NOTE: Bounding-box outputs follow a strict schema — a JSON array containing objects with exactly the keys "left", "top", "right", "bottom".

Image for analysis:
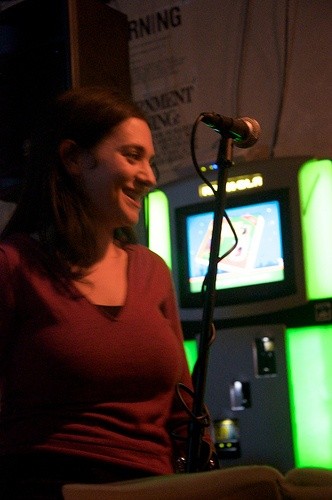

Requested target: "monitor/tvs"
[{"left": 174, "top": 186, "right": 296, "bottom": 309}]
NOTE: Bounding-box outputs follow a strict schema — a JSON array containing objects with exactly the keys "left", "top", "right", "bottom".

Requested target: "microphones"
[{"left": 199, "top": 111, "right": 261, "bottom": 148}]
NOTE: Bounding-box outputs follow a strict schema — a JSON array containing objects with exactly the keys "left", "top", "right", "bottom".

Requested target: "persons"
[{"left": 0, "top": 90, "right": 213, "bottom": 497}]
